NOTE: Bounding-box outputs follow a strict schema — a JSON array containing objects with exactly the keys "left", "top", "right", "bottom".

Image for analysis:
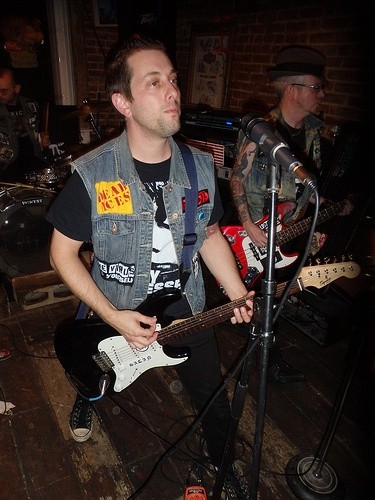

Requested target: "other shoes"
[{"left": 268, "top": 360, "right": 306, "bottom": 382}]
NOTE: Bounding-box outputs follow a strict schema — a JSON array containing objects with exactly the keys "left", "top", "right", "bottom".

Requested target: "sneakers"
[
  {"left": 224, "top": 468, "right": 249, "bottom": 500},
  {"left": 69, "top": 398, "right": 94, "bottom": 442}
]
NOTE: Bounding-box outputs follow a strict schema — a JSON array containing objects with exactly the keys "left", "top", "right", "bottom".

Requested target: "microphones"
[{"left": 241, "top": 113, "right": 313, "bottom": 187}]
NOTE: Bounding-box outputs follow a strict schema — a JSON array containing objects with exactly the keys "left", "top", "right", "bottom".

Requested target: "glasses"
[{"left": 292, "top": 82, "right": 322, "bottom": 93}]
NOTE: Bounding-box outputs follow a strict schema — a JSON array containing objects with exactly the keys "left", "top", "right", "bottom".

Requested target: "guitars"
[
  {"left": 55, "top": 253, "right": 363, "bottom": 399},
  {"left": 211, "top": 197, "right": 352, "bottom": 297}
]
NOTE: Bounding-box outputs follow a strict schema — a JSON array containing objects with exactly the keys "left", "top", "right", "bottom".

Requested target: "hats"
[{"left": 266, "top": 46, "right": 329, "bottom": 86}]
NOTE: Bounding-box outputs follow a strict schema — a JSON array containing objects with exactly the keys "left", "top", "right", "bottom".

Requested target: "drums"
[
  {"left": 0, "top": 185, "right": 61, "bottom": 280},
  {"left": 24, "top": 159, "right": 76, "bottom": 185}
]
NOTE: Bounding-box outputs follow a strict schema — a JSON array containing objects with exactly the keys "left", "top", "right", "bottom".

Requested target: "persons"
[
  {"left": 0, "top": 69, "right": 59, "bottom": 180},
  {"left": 233, "top": 62, "right": 361, "bottom": 383},
  {"left": 47, "top": 35, "right": 252, "bottom": 500}
]
